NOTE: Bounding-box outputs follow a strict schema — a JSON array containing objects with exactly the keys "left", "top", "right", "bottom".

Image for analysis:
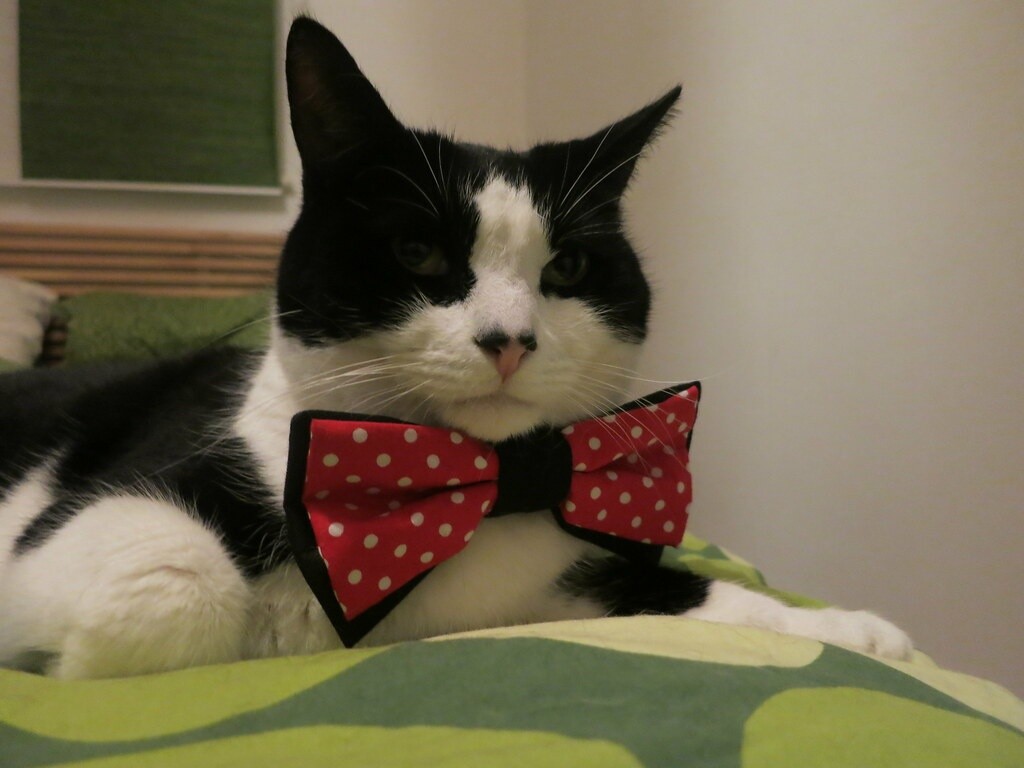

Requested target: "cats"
[{"left": 0, "top": 15, "right": 914, "bottom": 681}]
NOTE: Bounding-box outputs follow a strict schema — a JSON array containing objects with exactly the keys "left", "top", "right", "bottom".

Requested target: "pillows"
[{"left": 0, "top": 271, "right": 278, "bottom": 369}]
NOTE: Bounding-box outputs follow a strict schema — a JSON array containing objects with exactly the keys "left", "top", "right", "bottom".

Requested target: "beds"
[{"left": 0, "top": 222, "right": 1024, "bottom": 766}]
